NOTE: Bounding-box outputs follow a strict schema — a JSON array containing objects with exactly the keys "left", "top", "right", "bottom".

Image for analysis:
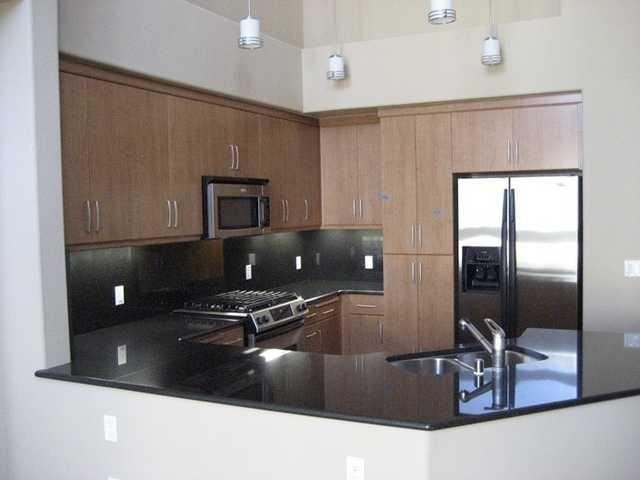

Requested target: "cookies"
[{"left": 201, "top": 175, "right": 272, "bottom": 241}]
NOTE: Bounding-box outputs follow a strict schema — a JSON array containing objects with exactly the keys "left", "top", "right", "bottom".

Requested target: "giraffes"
[{"left": 172, "top": 289, "right": 309, "bottom": 334}]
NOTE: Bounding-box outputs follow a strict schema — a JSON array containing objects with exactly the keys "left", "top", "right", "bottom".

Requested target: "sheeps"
[
  {"left": 459, "top": 371, "right": 509, "bottom": 410},
  {"left": 459, "top": 316, "right": 507, "bottom": 369}
]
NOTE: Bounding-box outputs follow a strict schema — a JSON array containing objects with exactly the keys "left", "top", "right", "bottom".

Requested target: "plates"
[
  {"left": 453, "top": 103, "right": 582, "bottom": 172},
  {"left": 261, "top": 112, "right": 296, "bottom": 232},
  {"left": 126, "top": 83, "right": 203, "bottom": 241},
  {"left": 203, "top": 99, "right": 261, "bottom": 180},
  {"left": 60, "top": 72, "right": 125, "bottom": 245},
  {"left": 381, "top": 256, "right": 456, "bottom": 350},
  {"left": 306, "top": 296, "right": 342, "bottom": 356},
  {"left": 321, "top": 125, "right": 383, "bottom": 227},
  {"left": 191, "top": 326, "right": 243, "bottom": 348},
  {"left": 382, "top": 113, "right": 455, "bottom": 256},
  {"left": 342, "top": 294, "right": 383, "bottom": 352}
]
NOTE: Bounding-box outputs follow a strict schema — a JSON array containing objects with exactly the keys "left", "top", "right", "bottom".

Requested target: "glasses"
[
  {"left": 479, "top": 0, "right": 503, "bottom": 69},
  {"left": 428, "top": 0, "right": 458, "bottom": 31},
  {"left": 326, "top": 0, "right": 347, "bottom": 81},
  {"left": 236, "top": 0, "right": 265, "bottom": 51}
]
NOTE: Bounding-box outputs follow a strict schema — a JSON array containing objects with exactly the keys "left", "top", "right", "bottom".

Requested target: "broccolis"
[
  {"left": 452, "top": 169, "right": 581, "bottom": 344},
  {"left": 454, "top": 344, "right": 581, "bottom": 410}
]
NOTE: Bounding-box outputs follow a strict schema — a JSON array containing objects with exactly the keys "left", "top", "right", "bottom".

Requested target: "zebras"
[{"left": 255, "top": 319, "right": 307, "bottom": 354}]
